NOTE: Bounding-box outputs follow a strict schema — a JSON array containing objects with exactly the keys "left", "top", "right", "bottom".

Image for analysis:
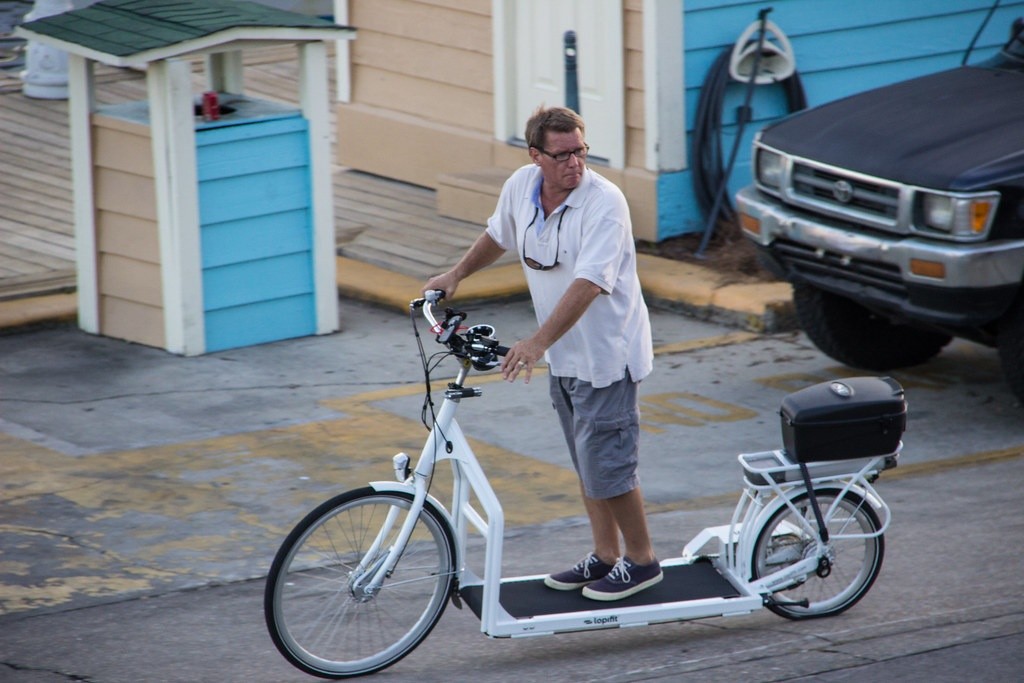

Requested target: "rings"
[{"left": 518, "top": 361, "right": 524, "bottom": 366}]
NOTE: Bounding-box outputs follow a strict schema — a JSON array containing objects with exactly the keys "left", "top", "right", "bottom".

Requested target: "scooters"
[{"left": 260, "top": 288, "right": 910, "bottom": 683}]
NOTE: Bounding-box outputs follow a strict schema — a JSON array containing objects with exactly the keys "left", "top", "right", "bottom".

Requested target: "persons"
[{"left": 423, "top": 106, "right": 665, "bottom": 600}]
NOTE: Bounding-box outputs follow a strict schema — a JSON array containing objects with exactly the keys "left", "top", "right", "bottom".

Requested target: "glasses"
[
  {"left": 529, "top": 143, "right": 589, "bottom": 162},
  {"left": 523, "top": 221, "right": 559, "bottom": 271}
]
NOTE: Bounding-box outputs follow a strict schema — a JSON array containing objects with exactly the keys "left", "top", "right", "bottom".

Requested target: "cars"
[{"left": 733, "top": 13, "right": 1024, "bottom": 402}]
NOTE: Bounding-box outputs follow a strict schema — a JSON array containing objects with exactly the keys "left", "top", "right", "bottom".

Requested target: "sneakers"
[
  {"left": 544, "top": 551, "right": 623, "bottom": 590},
  {"left": 582, "top": 555, "right": 664, "bottom": 601}
]
